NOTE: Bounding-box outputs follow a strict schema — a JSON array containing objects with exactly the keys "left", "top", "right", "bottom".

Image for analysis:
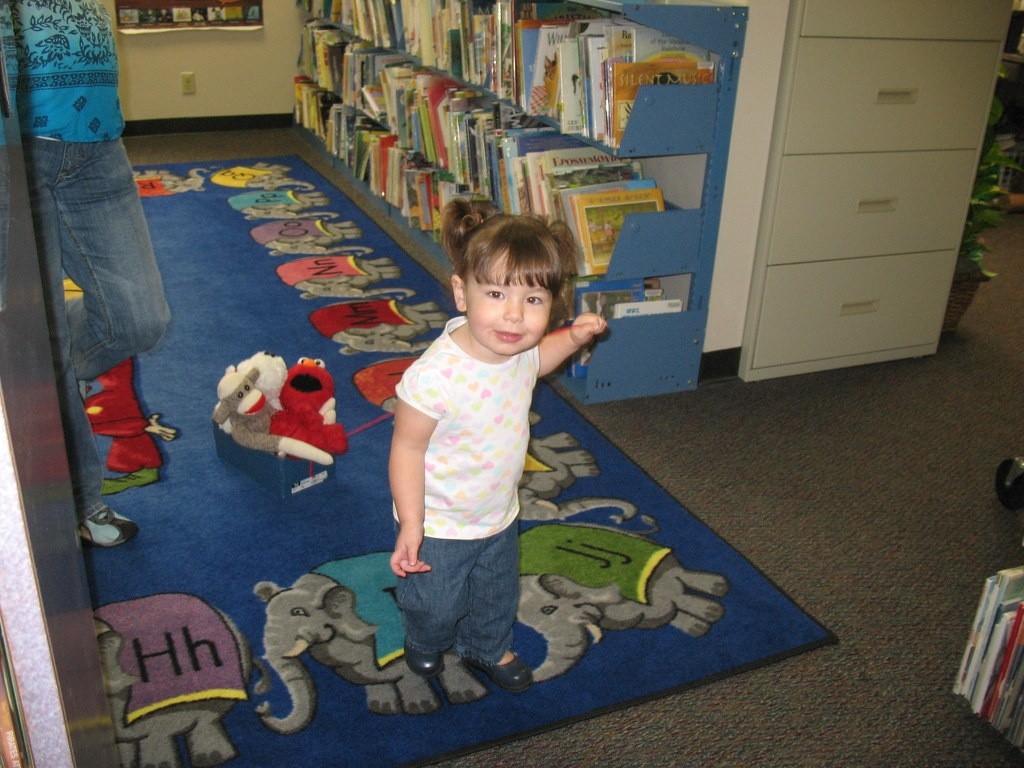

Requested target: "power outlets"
[{"left": 180, "top": 70, "right": 195, "bottom": 94}]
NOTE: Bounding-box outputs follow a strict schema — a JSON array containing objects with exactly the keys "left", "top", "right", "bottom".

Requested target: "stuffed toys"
[{"left": 216, "top": 349, "right": 349, "bottom": 465}]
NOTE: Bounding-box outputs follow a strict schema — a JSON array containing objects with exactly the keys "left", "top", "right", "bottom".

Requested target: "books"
[
  {"left": 293, "top": 75, "right": 475, "bottom": 243},
  {"left": 296, "top": 1, "right": 718, "bottom": 149},
  {"left": 445, "top": 109, "right": 683, "bottom": 379},
  {"left": 117, "top": 6, "right": 259, "bottom": 24}
]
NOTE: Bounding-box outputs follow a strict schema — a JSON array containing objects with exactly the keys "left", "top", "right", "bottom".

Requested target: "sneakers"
[{"left": 78, "top": 507, "right": 140, "bottom": 546}]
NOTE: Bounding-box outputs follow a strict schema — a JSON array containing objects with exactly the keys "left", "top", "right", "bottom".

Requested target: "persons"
[
  {"left": 387, "top": 200, "right": 608, "bottom": 693},
  {"left": 0, "top": 1, "right": 173, "bottom": 547}
]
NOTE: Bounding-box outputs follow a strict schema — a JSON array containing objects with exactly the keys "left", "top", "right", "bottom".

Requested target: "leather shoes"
[
  {"left": 461, "top": 650, "right": 534, "bottom": 692},
  {"left": 404, "top": 636, "right": 445, "bottom": 678}
]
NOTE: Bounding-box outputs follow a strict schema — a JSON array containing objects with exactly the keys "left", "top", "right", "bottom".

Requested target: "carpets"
[{"left": 63, "top": 152, "right": 846, "bottom": 768}]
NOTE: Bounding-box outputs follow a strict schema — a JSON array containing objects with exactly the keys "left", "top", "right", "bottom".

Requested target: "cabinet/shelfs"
[{"left": 288, "top": 0, "right": 1017, "bottom": 406}]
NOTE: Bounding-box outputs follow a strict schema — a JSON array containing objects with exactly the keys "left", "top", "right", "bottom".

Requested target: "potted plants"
[{"left": 938, "top": 63, "right": 1024, "bottom": 332}]
